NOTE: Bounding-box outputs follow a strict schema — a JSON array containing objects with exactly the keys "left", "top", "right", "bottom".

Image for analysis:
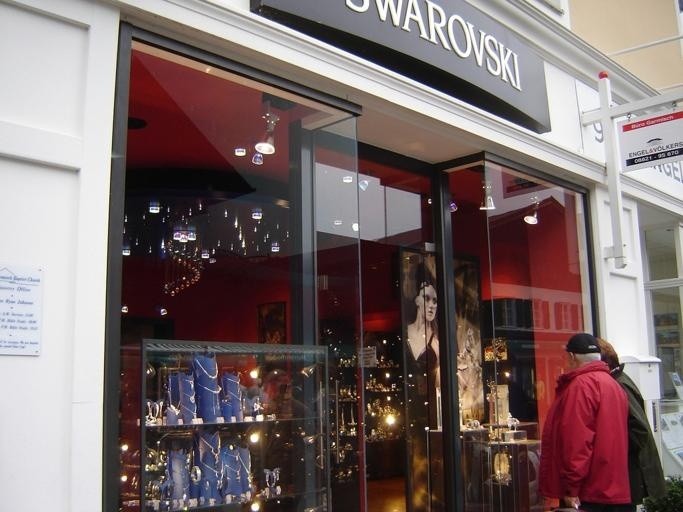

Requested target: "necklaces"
[{"left": 161, "top": 357, "right": 257, "bottom": 485}]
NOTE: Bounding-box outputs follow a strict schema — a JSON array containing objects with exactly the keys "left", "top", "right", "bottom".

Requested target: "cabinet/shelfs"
[
  {"left": 118, "top": 338, "right": 332, "bottom": 512},
  {"left": 364, "top": 332, "right": 407, "bottom": 480}
]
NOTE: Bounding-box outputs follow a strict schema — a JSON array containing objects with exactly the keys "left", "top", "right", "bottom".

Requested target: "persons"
[
  {"left": 408, "top": 272, "right": 446, "bottom": 461},
  {"left": 538, "top": 332, "right": 636, "bottom": 511},
  {"left": 594, "top": 337, "right": 664, "bottom": 512}
]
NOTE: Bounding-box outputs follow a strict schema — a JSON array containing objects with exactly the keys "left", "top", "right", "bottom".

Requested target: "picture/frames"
[{"left": 257, "top": 301, "right": 286, "bottom": 370}]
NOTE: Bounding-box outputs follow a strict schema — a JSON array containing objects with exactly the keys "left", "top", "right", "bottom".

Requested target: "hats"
[{"left": 566, "top": 333, "right": 601, "bottom": 353}]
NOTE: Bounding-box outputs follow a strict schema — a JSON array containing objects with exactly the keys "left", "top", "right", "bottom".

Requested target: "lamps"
[
  {"left": 524, "top": 194, "right": 540, "bottom": 225},
  {"left": 255, "top": 90, "right": 296, "bottom": 156},
  {"left": 468, "top": 164, "right": 496, "bottom": 210}
]
